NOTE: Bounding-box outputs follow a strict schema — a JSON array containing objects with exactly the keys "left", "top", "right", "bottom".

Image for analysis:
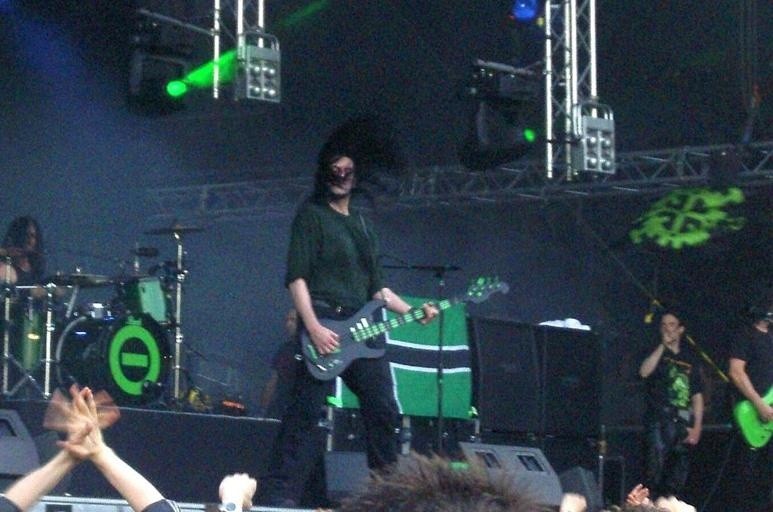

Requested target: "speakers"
[
  {"left": 458, "top": 440, "right": 564, "bottom": 507},
  {"left": 469, "top": 316, "right": 540, "bottom": 435},
  {"left": 0, "top": 408, "right": 41, "bottom": 477},
  {"left": 561, "top": 467, "right": 603, "bottom": 511},
  {"left": 536, "top": 324, "right": 603, "bottom": 437},
  {"left": 309, "top": 449, "right": 369, "bottom": 506}
]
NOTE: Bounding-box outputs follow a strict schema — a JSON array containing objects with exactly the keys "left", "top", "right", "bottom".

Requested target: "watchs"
[{"left": 219, "top": 501, "right": 241, "bottom": 512}]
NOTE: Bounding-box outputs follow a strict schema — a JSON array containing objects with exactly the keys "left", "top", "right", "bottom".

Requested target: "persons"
[
  {"left": 331, "top": 439, "right": 553, "bottom": 512},
  {"left": 252, "top": 105, "right": 442, "bottom": 512},
  {"left": 559, "top": 483, "right": 696, "bottom": 511},
  {"left": 0, "top": 214, "right": 103, "bottom": 391},
  {"left": 632, "top": 308, "right": 707, "bottom": 499},
  {"left": 723, "top": 284, "right": 773, "bottom": 511},
  {"left": 1, "top": 380, "right": 181, "bottom": 510},
  {"left": 216, "top": 471, "right": 256, "bottom": 512}
]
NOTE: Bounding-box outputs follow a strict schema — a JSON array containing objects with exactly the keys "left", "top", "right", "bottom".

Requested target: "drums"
[
  {"left": 55, "top": 310, "right": 170, "bottom": 406},
  {"left": 124, "top": 276, "right": 172, "bottom": 325},
  {"left": 9, "top": 303, "right": 44, "bottom": 370}
]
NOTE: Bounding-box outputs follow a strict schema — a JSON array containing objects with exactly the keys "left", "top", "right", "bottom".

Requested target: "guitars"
[
  {"left": 734, "top": 386, "right": 773, "bottom": 449},
  {"left": 300, "top": 276, "right": 509, "bottom": 381}
]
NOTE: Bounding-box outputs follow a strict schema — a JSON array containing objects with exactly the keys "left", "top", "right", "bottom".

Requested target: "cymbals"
[
  {"left": 44, "top": 273, "right": 111, "bottom": 287},
  {"left": 143, "top": 228, "right": 205, "bottom": 234},
  {"left": 0, "top": 246, "right": 48, "bottom": 257}
]
